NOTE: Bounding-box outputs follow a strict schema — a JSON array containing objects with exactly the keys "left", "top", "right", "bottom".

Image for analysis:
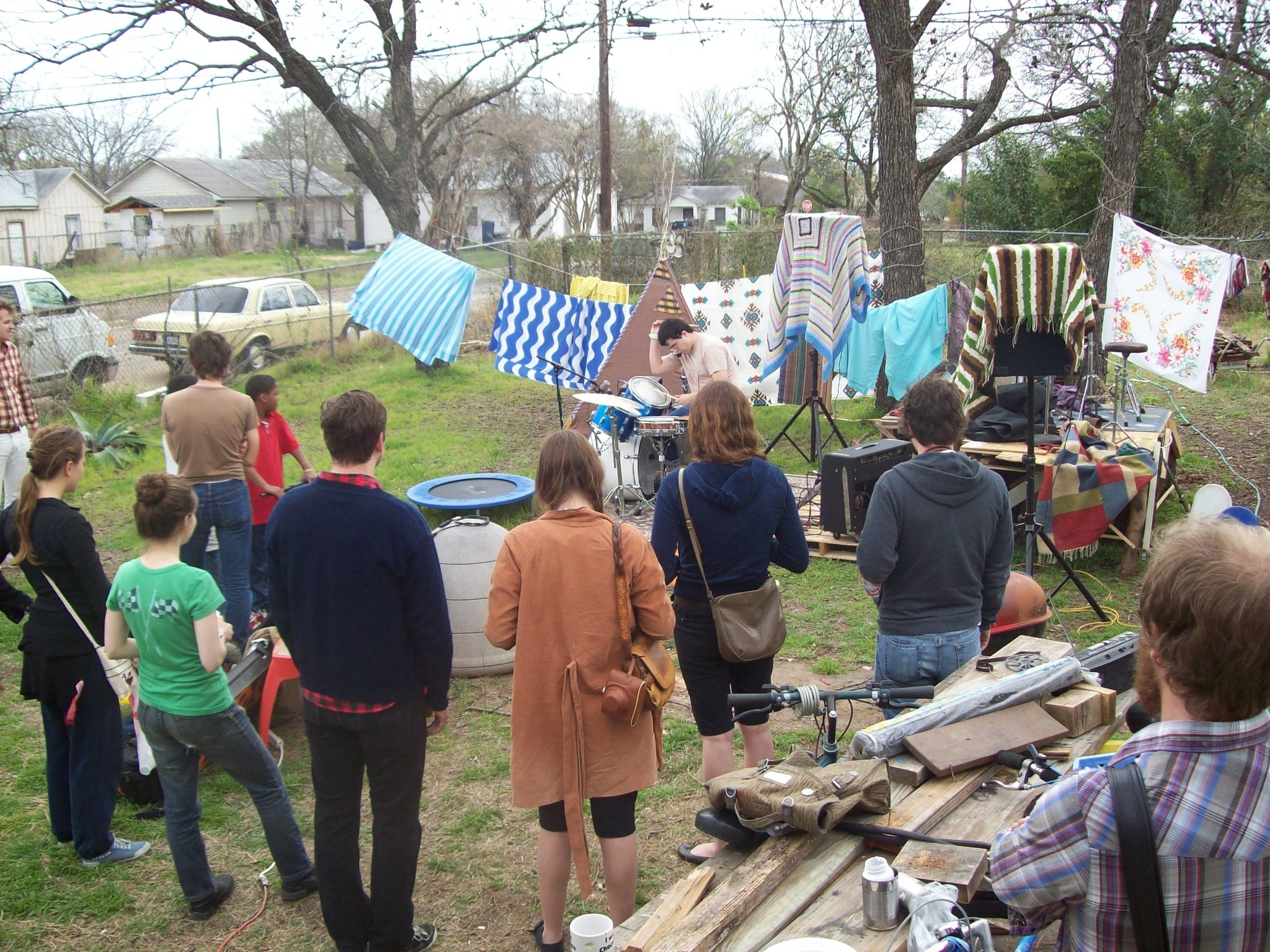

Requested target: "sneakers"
[
  {"left": 57, "top": 841, "right": 75, "bottom": 847},
  {"left": 82, "top": 833, "right": 151, "bottom": 867},
  {"left": 404, "top": 922, "right": 437, "bottom": 952}
]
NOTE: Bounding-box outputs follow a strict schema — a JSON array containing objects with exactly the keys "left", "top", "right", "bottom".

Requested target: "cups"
[
  {"left": 862, "top": 865, "right": 900, "bottom": 932},
  {"left": 569, "top": 913, "right": 614, "bottom": 952}
]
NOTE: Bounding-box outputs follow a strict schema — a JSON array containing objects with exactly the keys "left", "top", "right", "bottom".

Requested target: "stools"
[{"left": 1106, "top": 342, "right": 1148, "bottom": 421}]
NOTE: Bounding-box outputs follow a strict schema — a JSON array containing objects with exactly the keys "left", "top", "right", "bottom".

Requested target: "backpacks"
[{"left": 119, "top": 714, "right": 165, "bottom": 819}]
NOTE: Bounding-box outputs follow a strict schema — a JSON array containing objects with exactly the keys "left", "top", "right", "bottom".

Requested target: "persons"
[
  {"left": 264, "top": 389, "right": 454, "bottom": 952},
  {"left": 650, "top": 381, "right": 809, "bottom": 862},
  {"left": 161, "top": 375, "right": 223, "bottom": 615},
  {"left": 104, "top": 473, "right": 317, "bottom": 921},
  {"left": 160, "top": 329, "right": 259, "bottom": 656},
  {"left": 0, "top": 423, "right": 151, "bottom": 868},
  {"left": 988, "top": 513, "right": 1270, "bottom": 952},
  {"left": 648, "top": 319, "right": 739, "bottom": 471},
  {"left": 482, "top": 428, "right": 676, "bottom": 952},
  {"left": 856, "top": 378, "right": 1014, "bottom": 720},
  {"left": 0, "top": 297, "right": 39, "bottom": 569},
  {"left": 243, "top": 374, "right": 317, "bottom": 613}
]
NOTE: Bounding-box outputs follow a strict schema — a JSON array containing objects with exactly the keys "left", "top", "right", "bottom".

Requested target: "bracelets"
[{"left": 648, "top": 333, "right": 658, "bottom": 339}]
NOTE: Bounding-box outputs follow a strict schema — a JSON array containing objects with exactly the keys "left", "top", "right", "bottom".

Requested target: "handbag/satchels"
[
  {"left": 96, "top": 646, "right": 138, "bottom": 701},
  {"left": 128, "top": 672, "right": 157, "bottom": 775},
  {"left": 703, "top": 743, "right": 891, "bottom": 837},
  {"left": 612, "top": 522, "right": 675, "bottom": 712},
  {"left": 601, "top": 669, "right": 647, "bottom": 727},
  {"left": 711, "top": 577, "right": 786, "bottom": 664}
]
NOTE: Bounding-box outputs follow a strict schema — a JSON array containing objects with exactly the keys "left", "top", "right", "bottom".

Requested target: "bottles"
[{"left": 862, "top": 856, "right": 894, "bottom": 881}]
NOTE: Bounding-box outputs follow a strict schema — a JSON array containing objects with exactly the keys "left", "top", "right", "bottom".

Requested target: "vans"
[{"left": 0, "top": 263, "right": 121, "bottom": 398}]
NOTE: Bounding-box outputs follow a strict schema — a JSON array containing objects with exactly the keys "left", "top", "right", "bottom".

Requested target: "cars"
[{"left": 128, "top": 277, "right": 371, "bottom": 378}]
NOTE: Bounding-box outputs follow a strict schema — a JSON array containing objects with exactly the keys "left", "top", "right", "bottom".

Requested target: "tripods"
[
  {"left": 758, "top": 345, "right": 851, "bottom": 467},
  {"left": 1009, "top": 376, "right": 1111, "bottom": 623},
  {"left": 600, "top": 406, "right": 655, "bottom": 518}
]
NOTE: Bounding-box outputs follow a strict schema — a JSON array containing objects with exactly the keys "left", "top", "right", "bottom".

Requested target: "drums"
[
  {"left": 589, "top": 395, "right": 641, "bottom": 440},
  {"left": 596, "top": 437, "right": 661, "bottom": 501},
  {"left": 635, "top": 416, "right": 690, "bottom": 437},
  {"left": 622, "top": 376, "right": 673, "bottom": 416}
]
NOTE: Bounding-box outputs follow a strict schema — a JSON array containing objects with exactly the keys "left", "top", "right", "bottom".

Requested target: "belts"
[{"left": 0, "top": 424, "right": 24, "bottom": 434}]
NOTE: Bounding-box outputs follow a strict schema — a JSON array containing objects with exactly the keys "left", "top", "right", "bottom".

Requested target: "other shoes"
[
  {"left": 280, "top": 864, "right": 320, "bottom": 900},
  {"left": 677, "top": 840, "right": 710, "bottom": 864},
  {"left": 187, "top": 874, "right": 233, "bottom": 921},
  {"left": 533, "top": 919, "right": 563, "bottom": 952}
]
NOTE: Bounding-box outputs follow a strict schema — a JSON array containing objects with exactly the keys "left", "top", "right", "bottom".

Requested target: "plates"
[{"left": 762, "top": 937, "right": 857, "bottom": 952}]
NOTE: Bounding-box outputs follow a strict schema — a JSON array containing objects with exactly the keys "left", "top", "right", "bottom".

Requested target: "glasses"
[{"left": 669, "top": 340, "right": 679, "bottom": 352}]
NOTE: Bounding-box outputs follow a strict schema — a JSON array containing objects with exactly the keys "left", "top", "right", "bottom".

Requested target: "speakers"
[
  {"left": 991, "top": 312, "right": 1079, "bottom": 377},
  {"left": 819, "top": 439, "right": 913, "bottom": 533}
]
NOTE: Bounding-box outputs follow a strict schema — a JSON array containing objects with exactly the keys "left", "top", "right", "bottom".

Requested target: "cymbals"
[{"left": 573, "top": 391, "right": 646, "bottom": 409}]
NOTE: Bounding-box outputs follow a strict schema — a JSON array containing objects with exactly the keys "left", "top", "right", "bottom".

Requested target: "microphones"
[{"left": 596, "top": 379, "right": 611, "bottom": 394}]
